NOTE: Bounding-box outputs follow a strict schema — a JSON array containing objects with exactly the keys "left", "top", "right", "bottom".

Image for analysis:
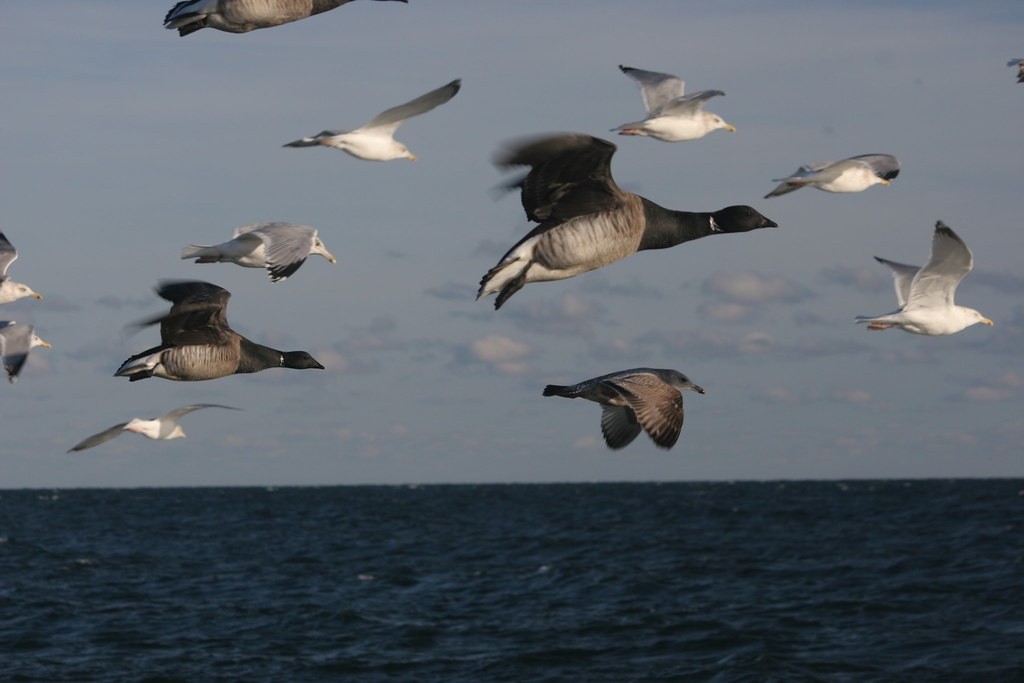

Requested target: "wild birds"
[
  {"left": 473, "top": 132, "right": 779, "bottom": 309},
  {"left": 66, "top": 403, "right": 246, "bottom": 454},
  {"left": 763, "top": 154, "right": 900, "bottom": 200},
  {"left": 281, "top": 78, "right": 461, "bottom": 162},
  {"left": 1006, "top": 57, "right": 1024, "bottom": 84},
  {"left": 0, "top": 231, "right": 44, "bottom": 304},
  {"left": 542, "top": 367, "right": 705, "bottom": 452},
  {"left": 114, "top": 277, "right": 325, "bottom": 382},
  {"left": 181, "top": 217, "right": 336, "bottom": 285},
  {"left": 854, "top": 221, "right": 994, "bottom": 335},
  {"left": 162, "top": 0, "right": 408, "bottom": 38},
  {"left": 0, "top": 320, "right": 53, "bottom": 384},
  {"left": 609, "top": 64, "right": 736, "bottom": 141}
]
